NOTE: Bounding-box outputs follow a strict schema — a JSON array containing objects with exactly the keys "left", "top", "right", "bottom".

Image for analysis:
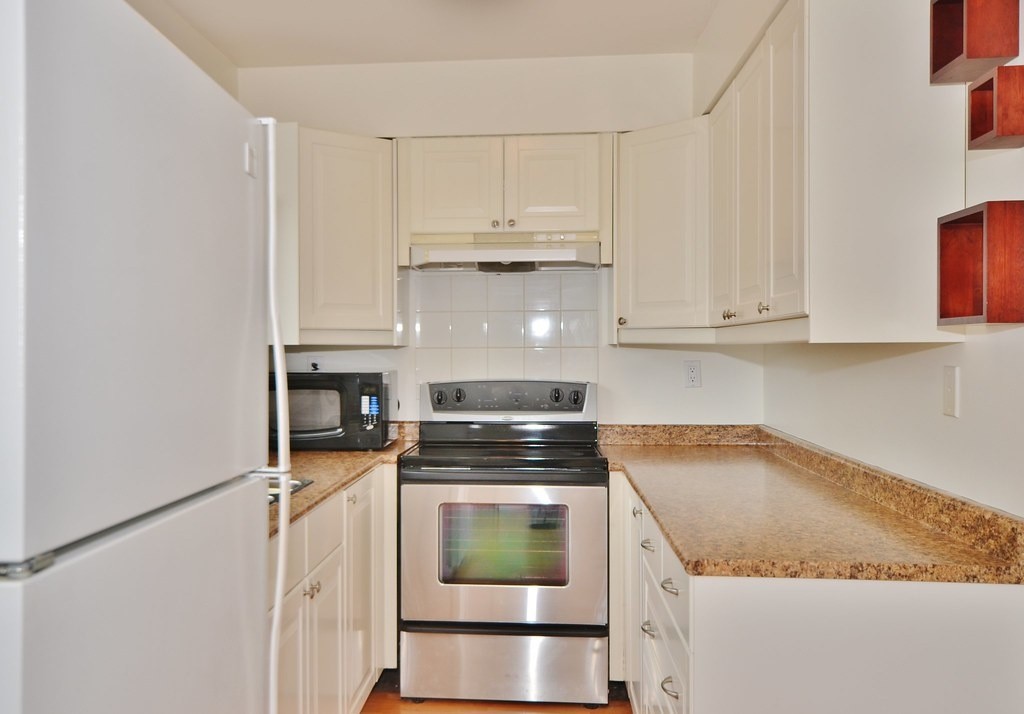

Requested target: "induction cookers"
[{"left": 399, "top": 380, "right": 610, "bottom": 470}]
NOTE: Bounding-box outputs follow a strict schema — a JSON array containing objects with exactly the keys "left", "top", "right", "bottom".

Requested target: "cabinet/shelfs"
[
  {"left": 758, "top": 0, "right": 966, "bottom": 344},
  {"left": 298, "top": 127, "right": 410, "bottom": 346},
  {"left": 344, "top": 470, "right": 374, "bottom": 714},
  {"left": 631, "top": 476, "right": 1024, "bottom": 714},
  {"left": 269, "top": 490, "right": 345, "bottom": 714},
  {"left": 707, "top": 30, "right": 766, "bottom": 344},
  {"left": 398, "top": 133, "right": 613, "bottom": 265},
  {"left": 615, "top": 113, "right": 710, "bottom": 344},
  {"left": 929, "top": 0, "right": 1024, "bottom": 327}
]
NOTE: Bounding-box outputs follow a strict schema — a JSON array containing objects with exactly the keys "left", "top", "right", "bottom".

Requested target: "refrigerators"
[{"left": 0, "top": 0, "right": 299, "bottom": 714}]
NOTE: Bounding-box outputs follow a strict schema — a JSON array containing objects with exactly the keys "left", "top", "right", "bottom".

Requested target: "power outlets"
[{"left": 683, "top": 360, "right": 701, "bottom": 388}]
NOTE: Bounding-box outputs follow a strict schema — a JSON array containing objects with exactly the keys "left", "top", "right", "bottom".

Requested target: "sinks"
[{"left": 265, "top": 475, "right": 314, "bottom": 505}]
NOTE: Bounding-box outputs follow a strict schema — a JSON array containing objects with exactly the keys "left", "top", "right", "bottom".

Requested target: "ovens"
[{"left": 397, "top": 473, "right": 610, "bottom": 629}]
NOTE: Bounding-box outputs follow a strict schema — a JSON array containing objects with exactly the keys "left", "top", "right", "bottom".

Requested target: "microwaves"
[{"left": 268, "top": 369, "right": 399, "bottom": 450}]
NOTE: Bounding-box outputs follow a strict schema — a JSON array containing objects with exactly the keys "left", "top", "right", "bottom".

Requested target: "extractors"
[{"left": 408, "top": 240, "right": 602, "bottom": 273}]
